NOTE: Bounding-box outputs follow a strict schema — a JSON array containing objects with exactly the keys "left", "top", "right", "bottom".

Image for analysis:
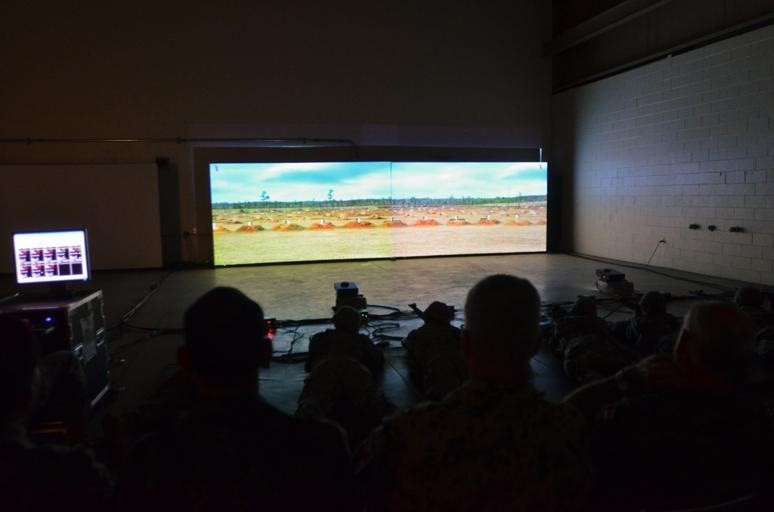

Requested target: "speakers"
[{"left": 0, "top": 288, "right": 114, "bottom": 415}]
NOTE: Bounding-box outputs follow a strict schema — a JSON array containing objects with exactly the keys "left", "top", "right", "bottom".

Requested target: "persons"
[
  {"left": 291, "top": 274, "right": 606, "bottom": 511},
  {"left": 530, "top": 275, "right": 772, "bottom": 510},
  {"left": 112, "top": 288, "right": 359, "bottom": 511},
  {"left": 2, "top": 316, "right": 115, "bottom": 508}
]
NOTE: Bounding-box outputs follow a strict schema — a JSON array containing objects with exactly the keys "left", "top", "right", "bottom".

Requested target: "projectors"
[
  {"left": 595, "top": 267, "right": 626, "bottom": 281},
  {"left": 334, "top": 281, "right": 359, "bottom": 294}
]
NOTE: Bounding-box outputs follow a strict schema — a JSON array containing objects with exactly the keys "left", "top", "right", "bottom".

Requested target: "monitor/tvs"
[{"left": 10, "top": 227, "right": 91, "bottom": 299}]
